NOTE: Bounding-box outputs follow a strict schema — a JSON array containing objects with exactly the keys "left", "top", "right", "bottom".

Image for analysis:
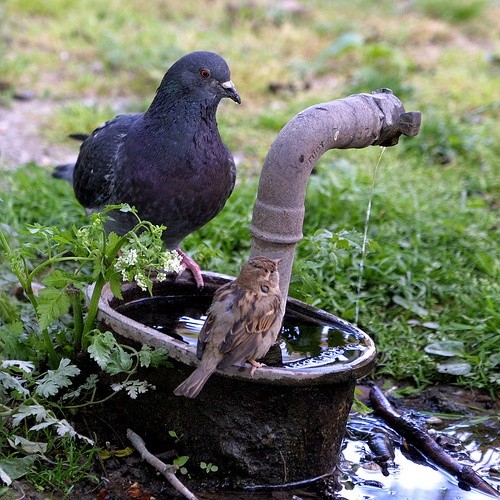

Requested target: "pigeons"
[{"left": 51, "top": 50, "right": 242, "bottom": 291}]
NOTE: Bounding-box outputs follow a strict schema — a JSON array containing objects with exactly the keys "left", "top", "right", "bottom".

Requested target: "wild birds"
[{"left": 172, "top": 254, "right": 286, "bottom": 399}]
